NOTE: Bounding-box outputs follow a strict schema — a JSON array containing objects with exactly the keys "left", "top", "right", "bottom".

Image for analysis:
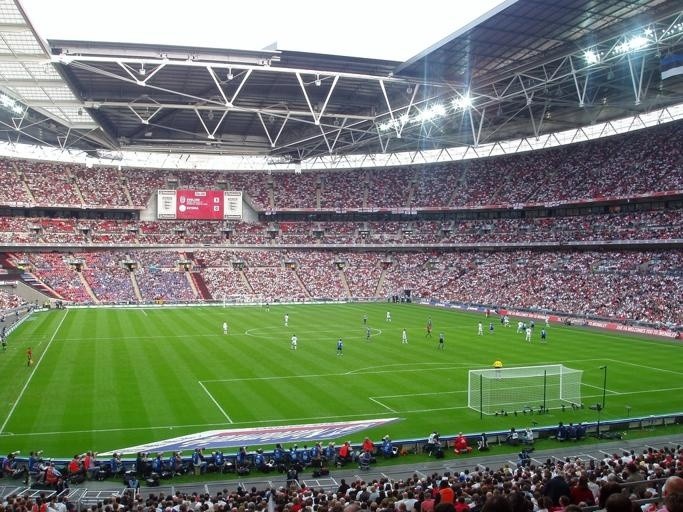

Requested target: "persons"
[
  {"left": 0, "top": 249, "right": 681, "bottom": 332},
  {"left": 1, "top": 156, "right": 682, "bottom": 210},
  {"left": 367, "top": 326, "right": 371, "bottom": 338},
  {"left": 25, "top": 347, "right": 34, "bottom": 368},
  {"left": 541, "top": 326, "right": 546, "bottom": 345},
  {"left": 335, "top": 338, "right": 343, "bottom": 355},
  {"left": 223, "top": 320, "right": 228, "bottom": 335},
  {"left": 285, "top": 313, "right": 288, "bottom": 329},
  {"left": 0, "top": 335, "right": 9, "bottom": 351},
  {"left": 401, "top": 327, "right": 408, "bottom": 344},
  {"left": 436, "top": 331, "right": 444, "bottom": 352},
  {"left": 363, "top": 313, "right": 367, "bottom": 325},
  {"left": 425, "top": 325, "right": 432, "bottom": 337},
  {"left": 0, "top": 214, "right": 683, "bottom": 244},
  {"left": 0, "top": 419, "right": 683, "bottom": 512},
  {"left": 266, "top": 302, "right": 269, "bottom": 312},
  {"left": 524, "top": 325, "right": 531, "bottom": 343},
  {"left": 491, "top": 358, "right": 502, "bottom": 381},
  {"left": 478, "top": 307, "right": 550, "bottom": 337},
  {"left": 386, "top": 310, "right": 392, "bottom": 322},
  {"left": 427, "top": 318, "right": 432, "bottom": 326},
  {"left": 288, "top": 334, "right": 297, "bottom": 351}
]
